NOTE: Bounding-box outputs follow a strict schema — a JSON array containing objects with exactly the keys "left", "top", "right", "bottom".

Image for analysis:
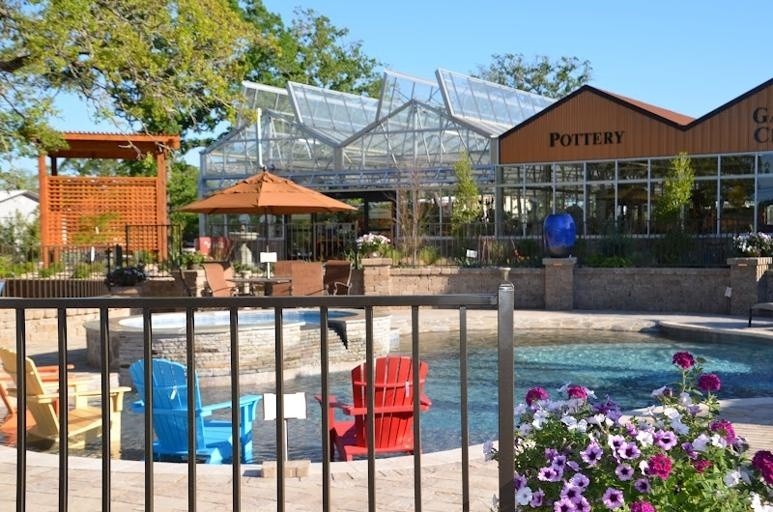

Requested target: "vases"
[
  {"left": 367, "top": 250, "right": 380, "bottom": 258},
  {"left": 544, "top": 212, "right": 577, "bottom": 258}
]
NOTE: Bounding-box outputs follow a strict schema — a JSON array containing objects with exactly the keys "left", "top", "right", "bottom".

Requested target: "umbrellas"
[{"left": 172, "top": 166, "right": 358, "bottom": 279}]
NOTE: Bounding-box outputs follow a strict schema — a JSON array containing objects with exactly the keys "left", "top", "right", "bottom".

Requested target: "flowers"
[
  {"left": 355, "top": 232, "right": 393, "bottom": 257},
  {"left": 733, "top": 224, "right": 773, "bottom": 257},
  {"left": 481, "top": 350, "right": 773, "bottom": 512}
]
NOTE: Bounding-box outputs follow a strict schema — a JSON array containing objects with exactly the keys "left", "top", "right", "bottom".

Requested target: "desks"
[{"left": 226, "top": 276, "right": 293, "bottom": 309}]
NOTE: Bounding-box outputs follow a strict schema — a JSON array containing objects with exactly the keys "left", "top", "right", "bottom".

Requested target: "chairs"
[
  {"left": 321, "top": 260, "right": 354, "bottom": 295},
  {"left": 0, "top": 347, "right": 263, "bottom": 466},
  {"left": 200, "top": 263, "right": 237, "bottom": 312},
  {"left": 314, "top": 355, "right": 433, "bottom": 463}
]
